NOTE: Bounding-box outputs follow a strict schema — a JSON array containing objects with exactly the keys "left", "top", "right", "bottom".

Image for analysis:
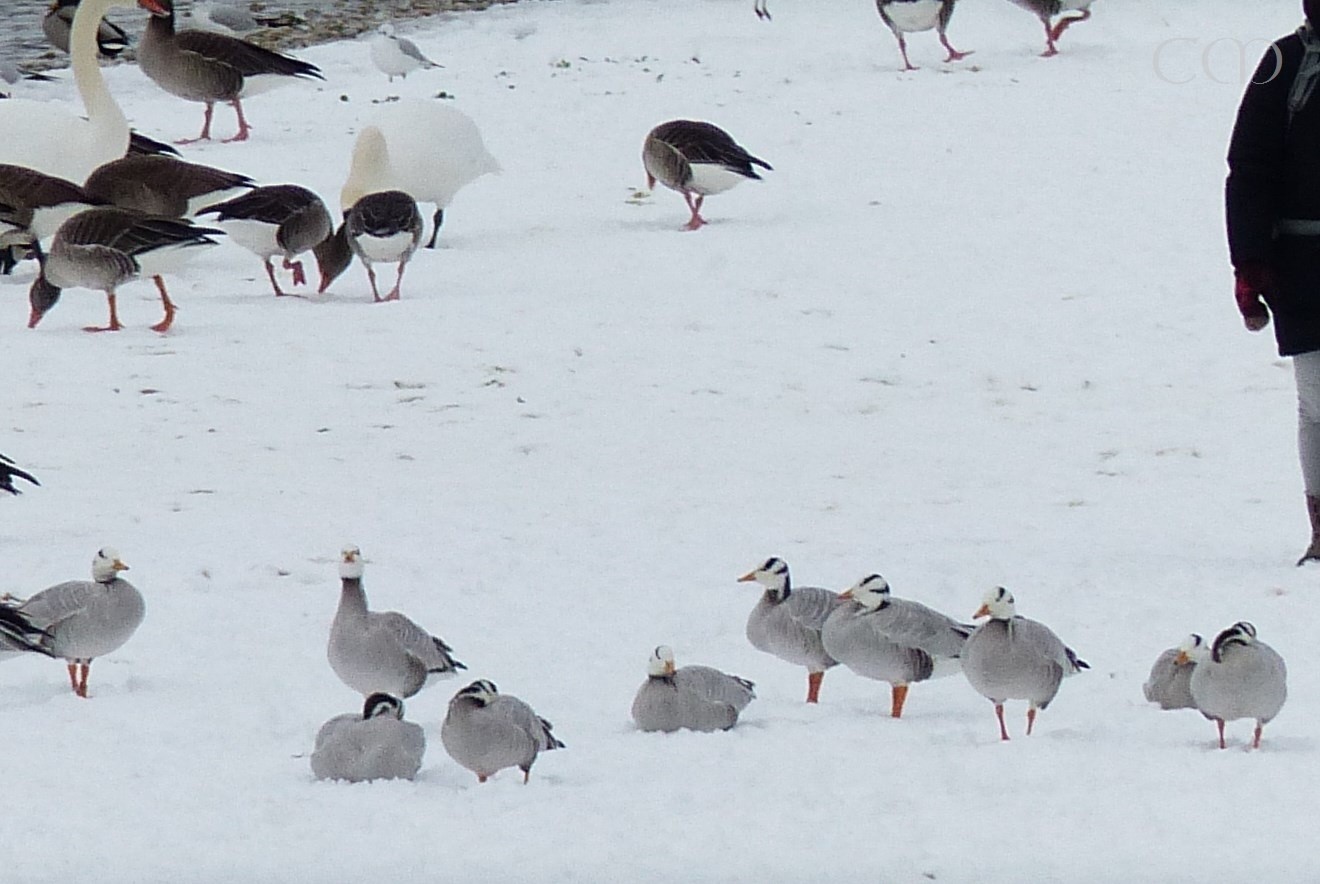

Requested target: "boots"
[{"left": 1298, "top": 495, "right": 1320, "bottom": 565}]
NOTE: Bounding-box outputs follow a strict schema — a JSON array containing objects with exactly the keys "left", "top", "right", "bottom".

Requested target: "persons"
[{"left": 1226, "top": 0, "right": 1320, "bottom": 566}]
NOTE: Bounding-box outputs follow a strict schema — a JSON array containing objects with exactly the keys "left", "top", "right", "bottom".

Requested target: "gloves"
[{"left": 1235, "top": 269, "right": 1269, "bottom": 330}]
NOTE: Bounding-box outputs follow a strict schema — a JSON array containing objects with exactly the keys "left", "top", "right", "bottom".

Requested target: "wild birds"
[
  {"left": 754, "top": 0, "right": 1095, "bottom": 71},
  {"left": 43, "top": 0, "right": 132, "bottom": 59},
  {"left": 959, "top": 587, "right": 1089, "bottom": 740},
  {"left": 192, "top": 185, "right": 333, "bottom": 298},
  {"left": 29, "top": 206, "right": 227, "bottom": 332},
  {"left": 643, "top": 120, "right": 774, "bottom": 232},
  {"left": 1189, "top": 622, "right": 1287, "bottom": 748},
  {"left": 0, "top": 454, "right": 145, "bottom": 696},
  {"left": 441, "top": 680, "right": 565, "bottom": 784},
  {"left": 339, "top": 24, "right": 503, "bottom": 249},
  {"left": 0, "top": 0, "right": 182, "bottom": 187},
  {"left": 1143, "top": 633, "right": 1212, "bottom": 709},
  {"left": 0, "top": 156, "right": 261, "bottom": 275},
  {"left": 631, "top": 645, "right": 757, "bottom": 733},
  {"left": 318, "top": 190, "right": 424, "bottom": 302},
  {"left": 134, "top": 0, "right": 327, "bottom": 144},
  {"left": 311, "top": 693, "right": 425, "bottom": 782},
  {"left": 821, "top": 574, "right": 976, "bottom": 717},
  {"left": 326, "top": 542, "right": 467, "bottom": 707},
  {"left": 737, "top": 557, "right": 853, "bottom": 702}
]
